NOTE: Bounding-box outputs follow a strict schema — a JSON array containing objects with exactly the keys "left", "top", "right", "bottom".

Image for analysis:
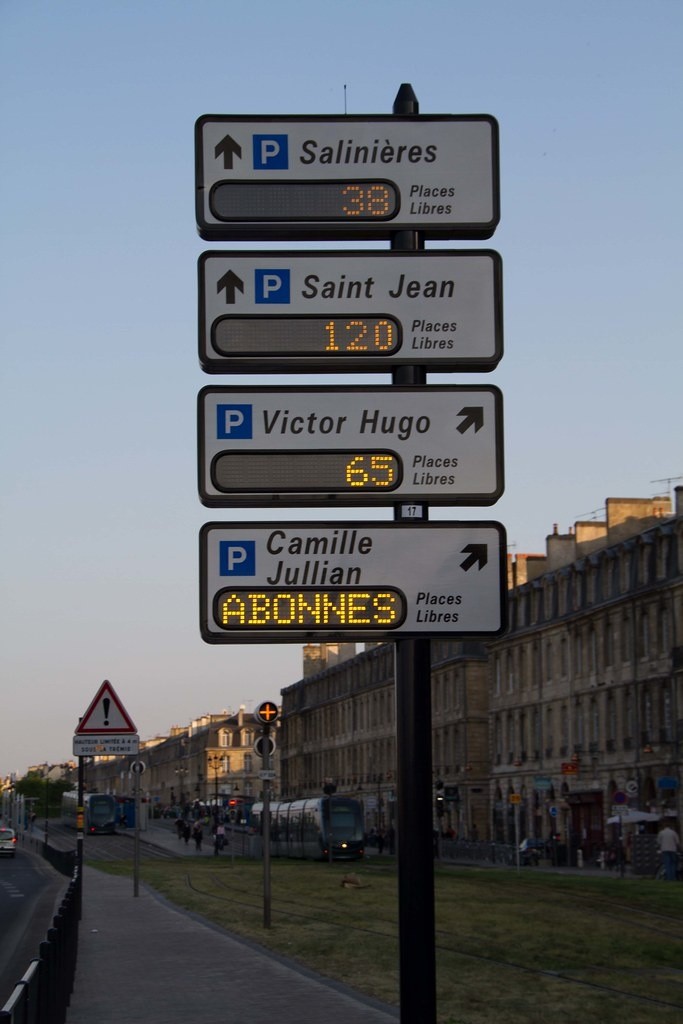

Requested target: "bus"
[
  {"left": 248, "top": 797, "right": 369, "bottom": 863},
  {"left": 61, "top": 790, "right": 120, "bottom": 837}
]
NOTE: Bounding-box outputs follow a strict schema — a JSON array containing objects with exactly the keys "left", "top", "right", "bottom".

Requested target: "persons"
[{"left": 144, "top": 801, "right": 683, "bottom": 882}]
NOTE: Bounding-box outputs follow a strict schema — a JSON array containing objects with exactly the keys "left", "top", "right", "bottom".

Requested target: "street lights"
[
  {"left": 172, "top": 764, "right": 190, "bottom": 815},
  {"left": 208, "top": 754, "right": 224, "bottom": 824}
]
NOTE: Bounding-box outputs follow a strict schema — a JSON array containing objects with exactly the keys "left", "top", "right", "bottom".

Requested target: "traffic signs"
[{"left": 194, "top": 111, "right": 511, "bottom": 648}]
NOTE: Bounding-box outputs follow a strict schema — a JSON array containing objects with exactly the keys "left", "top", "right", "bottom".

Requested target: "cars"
[
  {"left": 0, "top": 829, "right": 16, "bottom": 858},
  {"left": 519, "top": 837, "right": 565, "bottom": 866}
]
